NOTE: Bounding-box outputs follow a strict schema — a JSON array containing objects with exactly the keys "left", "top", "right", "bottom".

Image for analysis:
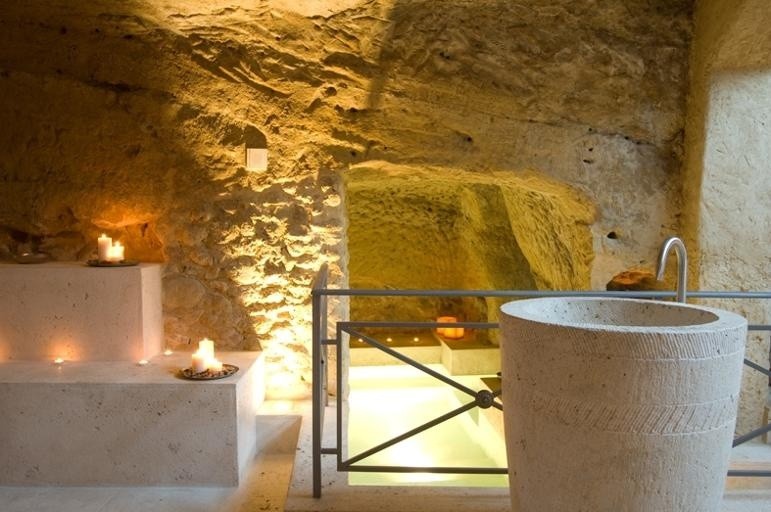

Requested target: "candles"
[
  {"left": 191, "top": 337, "right": 224, "bottom": 374},
  {"left": 97, "top": 233, "right": 125, "bottom": 262}
]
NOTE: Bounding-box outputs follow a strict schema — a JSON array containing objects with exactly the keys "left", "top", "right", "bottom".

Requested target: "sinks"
[{"left": 499, "top": 297, "right": 747, "bottom": 512}]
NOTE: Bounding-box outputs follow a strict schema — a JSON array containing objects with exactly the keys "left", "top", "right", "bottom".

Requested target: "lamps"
[{"left": 436, "top": 317, "right": 464, "bottom": 340}]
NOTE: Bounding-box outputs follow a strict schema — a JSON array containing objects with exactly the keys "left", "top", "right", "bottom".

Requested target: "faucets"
[{"left": 652, "top": 236, "right": 688, "bottom": 302}]
